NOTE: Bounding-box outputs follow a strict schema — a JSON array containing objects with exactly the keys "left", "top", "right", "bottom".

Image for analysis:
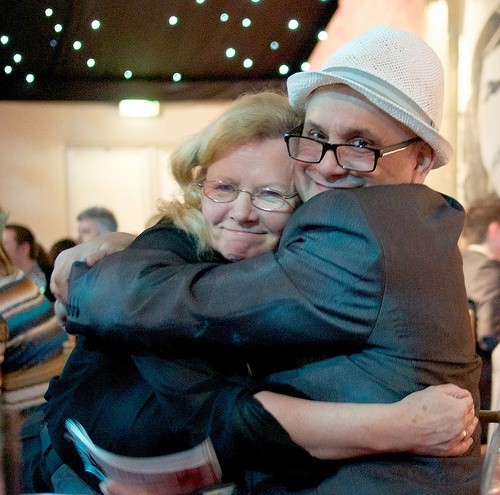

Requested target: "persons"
[
  {"left": 47, "top": 28, "right": 485, "bottom": 495},
  {"left": 0, "top": 87, "right": 500, "bottom": 495}
]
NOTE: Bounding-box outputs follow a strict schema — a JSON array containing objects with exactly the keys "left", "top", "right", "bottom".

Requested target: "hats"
[{"left": 286, "top": 26, "right": 455, "bottom": 169}]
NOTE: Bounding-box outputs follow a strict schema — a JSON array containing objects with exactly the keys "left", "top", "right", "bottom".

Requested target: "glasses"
[
  {"left": 284, "top": 122, "right": 422, "bottom": 172},
  {"left": 198, "top": 178, "right": 298, "bottom": 211}
]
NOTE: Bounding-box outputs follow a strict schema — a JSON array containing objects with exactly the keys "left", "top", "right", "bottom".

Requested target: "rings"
[{"left": 463, "top": 430, "right": 467, "bottom": 439}]
